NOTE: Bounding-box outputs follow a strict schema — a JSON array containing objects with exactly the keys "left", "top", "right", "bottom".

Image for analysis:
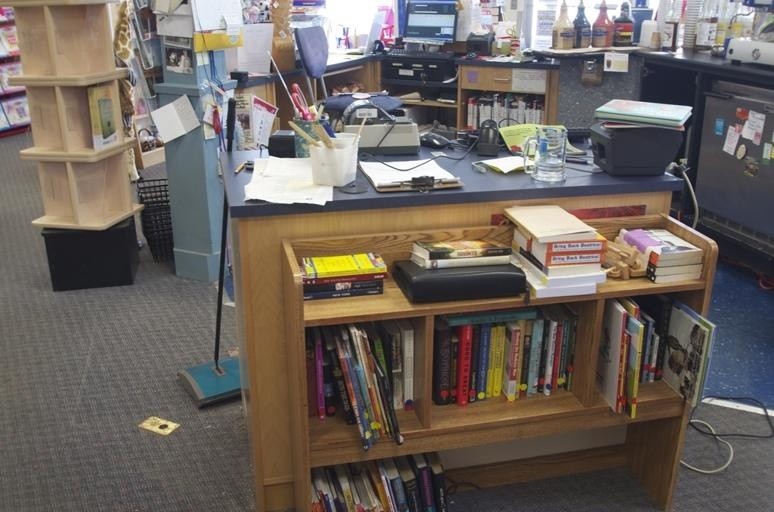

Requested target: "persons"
[{"left": 164, "top": 48, "right": 191, "bottom": 68}]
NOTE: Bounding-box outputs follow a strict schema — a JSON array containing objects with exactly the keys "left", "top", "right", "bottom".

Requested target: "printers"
[{"left": 589, "top": 122, "right": 684, "bottom": 176}]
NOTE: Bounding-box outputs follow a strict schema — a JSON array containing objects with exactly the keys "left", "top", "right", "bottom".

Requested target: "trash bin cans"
[{"left": 137, "top": 179, "right": 173, "bottom": 260}]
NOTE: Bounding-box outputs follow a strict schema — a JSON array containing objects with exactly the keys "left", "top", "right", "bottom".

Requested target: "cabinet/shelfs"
[
  {"left": 1, "top": 6, "right": 32, "bottom": 139},
  {"left": 455, "top": 53, "right": 559, "bottom": 138},
  {"left": 279, "top": 212, "right": 721, "bottom": 510}
]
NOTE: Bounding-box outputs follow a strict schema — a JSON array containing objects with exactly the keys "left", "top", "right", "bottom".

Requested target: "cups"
[{"left": 524, "top": 127, "right": 568, "bottom": 181}]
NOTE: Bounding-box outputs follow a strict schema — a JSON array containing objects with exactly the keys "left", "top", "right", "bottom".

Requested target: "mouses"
[{"left": 420, "top": 132, "right": 449, "bottom": 149}]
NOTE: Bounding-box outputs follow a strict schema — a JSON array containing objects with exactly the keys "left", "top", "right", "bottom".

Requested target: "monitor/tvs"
[{"left": 403, "top": 0, "right": 458, "bottom": 52}]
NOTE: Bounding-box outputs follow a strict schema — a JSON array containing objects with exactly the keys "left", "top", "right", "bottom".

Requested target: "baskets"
[{"left": 137, "top": 179, "right": 174, "bottom": 262}]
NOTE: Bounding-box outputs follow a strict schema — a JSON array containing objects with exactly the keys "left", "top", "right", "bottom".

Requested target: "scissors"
[
  {"left": 507, "top": 24, "right": 519, "bottom": 40},
  {"left": 291, "top": 83, "right": 311, "bottom": 121}
]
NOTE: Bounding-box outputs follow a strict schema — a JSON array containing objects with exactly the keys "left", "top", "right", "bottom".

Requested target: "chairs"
[{"left": 294, "top": 25, "right": 406, "bottom": 133}]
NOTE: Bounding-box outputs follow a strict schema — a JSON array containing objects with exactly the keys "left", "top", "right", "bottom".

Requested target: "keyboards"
[{"left": 388, "top": 49, "right": 453, "bottom": 59}]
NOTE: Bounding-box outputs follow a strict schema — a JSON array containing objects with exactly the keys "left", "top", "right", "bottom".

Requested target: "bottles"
[{"left": 551, "top": 1, "right": 633, "bottom": 49}]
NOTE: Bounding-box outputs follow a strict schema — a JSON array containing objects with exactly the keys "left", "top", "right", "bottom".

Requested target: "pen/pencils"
[
  {"left": 343, "top": 27, "right": 348, "bottom": 49},
  {"left": 323, "top": 123, "right": 338, "bottom": 139},
  {"left": 307, "top": 101, "right": 325, "bottom": 121},
  {"left": 234, "top": 160, "right": 248, "bottom": 173}
]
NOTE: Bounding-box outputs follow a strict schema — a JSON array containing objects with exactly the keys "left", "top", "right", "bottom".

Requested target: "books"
[
  {"left": 304, "top": 296, "right": 715, "bottom": 453},
  {"left": 87, "top": 85, "right": 123, "bottom": 153},
  {"left": 594, "top": 99, "right": 692, "bottom": 133},
  {"left": 497, "top": 124, "right": 587, "bottom": 158},
  {"left": 0, "top": 7, "right": 31, "bottom": 135},
  {"left": 311, "top": 453, "right": 449, "bottom": 512},
  {"left": 298, "top": 203, "right": 704, "bottom": 299},
  {"left": 468, "top": 92, "right": 544, "bottom": 129}
]
